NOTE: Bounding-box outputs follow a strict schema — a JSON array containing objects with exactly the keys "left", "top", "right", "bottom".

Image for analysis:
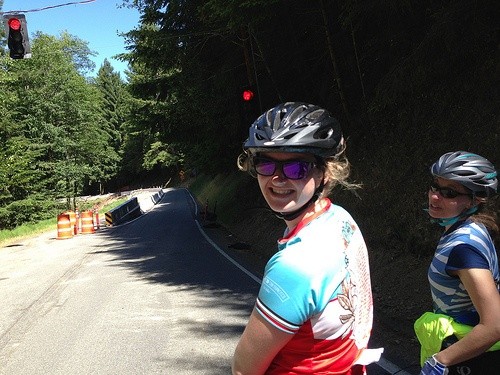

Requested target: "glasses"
[
  {"left": 252, "top": 156, "right": 319, "bottom": 180},
  {"left": 429, "top": 182, "right": 467, "bottom": 198}
]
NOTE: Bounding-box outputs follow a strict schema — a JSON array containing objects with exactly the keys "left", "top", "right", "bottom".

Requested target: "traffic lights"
[
  {"left": 7, "top": 17, "right": 25, "bottom": 59},
  {"left": 241, "top": 88, "right": 257, "bottom": 104}
]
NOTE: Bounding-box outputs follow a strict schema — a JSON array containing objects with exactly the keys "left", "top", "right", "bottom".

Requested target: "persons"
[
  {"left": 231, "top": 103, "right": 374, "bottom": 375},
  {"left": 419, "top": 151, "right": 500, "bottom": 375}
]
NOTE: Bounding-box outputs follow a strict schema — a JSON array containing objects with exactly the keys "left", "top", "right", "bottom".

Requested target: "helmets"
[
  {"left": 243, "top": 101, "right": 344, "bottom": 157},
  {"left": 431, "top": 151, "right": 497, "bottom": 193}
]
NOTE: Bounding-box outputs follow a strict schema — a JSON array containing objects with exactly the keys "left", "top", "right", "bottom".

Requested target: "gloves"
[{"left": 419, "top": 353, "right": 449, "bottom": 375}]
